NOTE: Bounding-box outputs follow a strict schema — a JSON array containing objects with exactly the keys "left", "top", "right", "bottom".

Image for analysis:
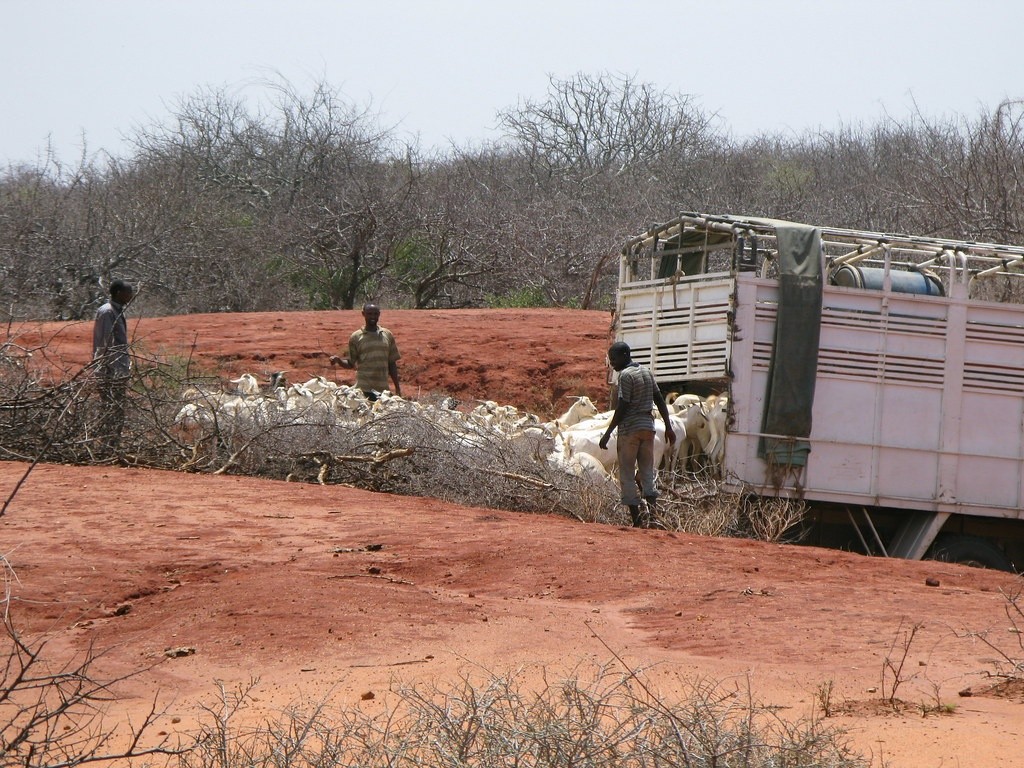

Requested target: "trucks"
[{"left": 606, "top": 211, "right": 1024, "bottom": 574}]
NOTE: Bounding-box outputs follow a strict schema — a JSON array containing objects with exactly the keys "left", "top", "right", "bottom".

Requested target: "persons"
[
  {"left": 93, "top": 279, "right": 132, "bottom": 456},
  {"left": 329, "top": 305, "right": 401, "bottom": 401},
  {"left": 599, "top": 342, "right": 676, "bottom": 529}
]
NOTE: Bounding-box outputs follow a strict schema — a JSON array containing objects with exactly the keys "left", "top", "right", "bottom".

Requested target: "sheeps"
[{"left": 174, "top": 371, "right": 728, "bottom": 488}]
